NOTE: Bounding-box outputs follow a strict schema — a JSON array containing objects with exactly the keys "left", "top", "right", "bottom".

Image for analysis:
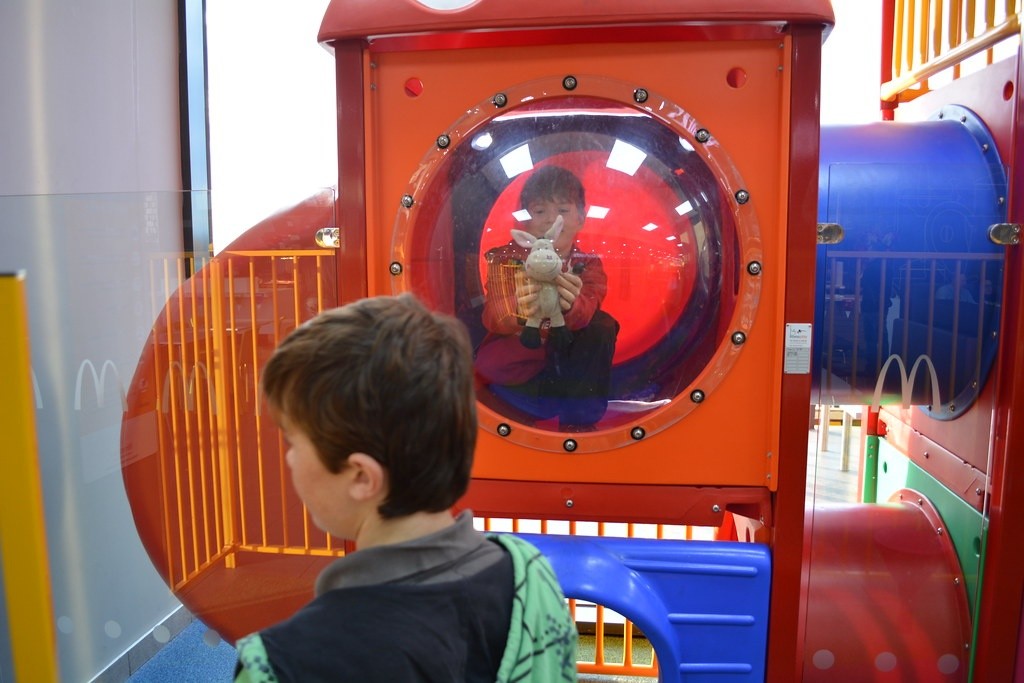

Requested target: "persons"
[
  {"left": 475, "top": 167, "right": 621, "bottom": 434},
  {"left": 220, "top": 294, "right": 579, "bottom": 683}
]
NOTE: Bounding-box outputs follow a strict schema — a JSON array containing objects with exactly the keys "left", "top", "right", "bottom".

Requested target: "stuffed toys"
[{"left": 510, "top": 214, "right": 583, "bottom": 350}]
